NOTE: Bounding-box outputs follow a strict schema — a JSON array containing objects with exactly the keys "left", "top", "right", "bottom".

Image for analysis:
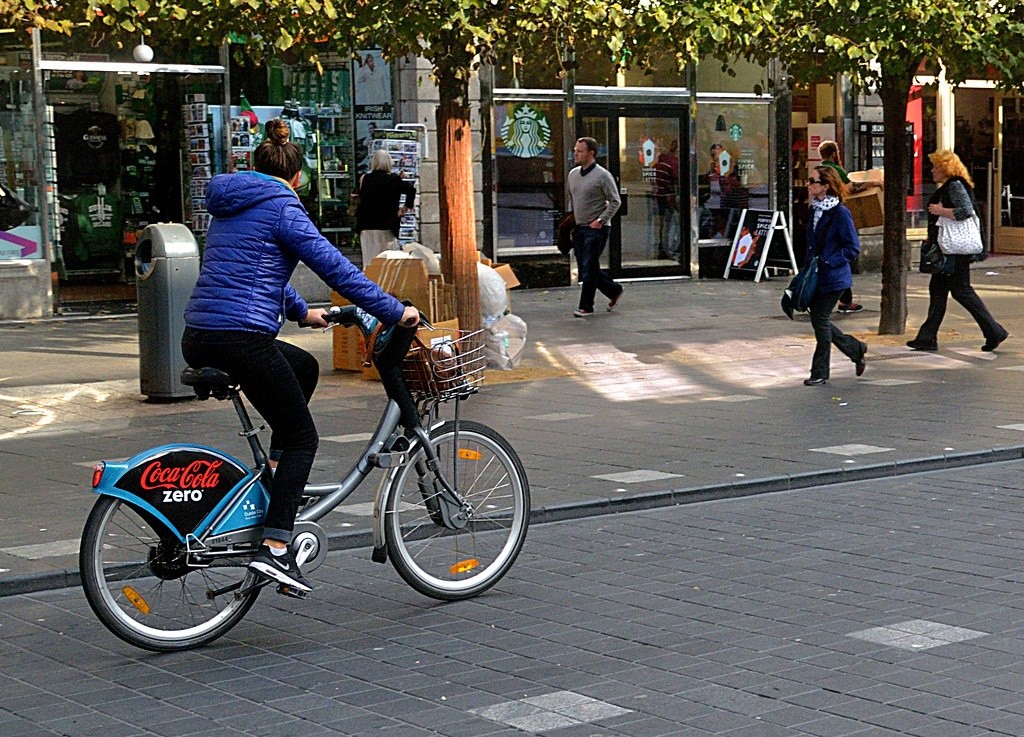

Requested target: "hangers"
[
  {"left": 83, "top": 183, "right": 107, "bottom": 196},
  {"left": 84, "top": 103, "right": 101, "bottom": 112}
]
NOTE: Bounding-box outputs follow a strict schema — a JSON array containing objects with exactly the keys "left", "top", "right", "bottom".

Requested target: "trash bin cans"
[{"left": 136, "top": 222, "right": 198, "bottom": 400}]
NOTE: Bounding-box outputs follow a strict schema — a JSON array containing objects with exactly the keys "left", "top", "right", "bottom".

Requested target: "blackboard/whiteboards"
[{"left": 724, "top": 209, "right": 798, "bottom": 271}]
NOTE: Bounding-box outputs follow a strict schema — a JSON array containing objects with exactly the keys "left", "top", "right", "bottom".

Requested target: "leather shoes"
[
  {"left": 804, "top": 376, "right": 829, "bottom": 385},
  {"left": 856, "top": 343, "right": 868, "bottom": 376}
]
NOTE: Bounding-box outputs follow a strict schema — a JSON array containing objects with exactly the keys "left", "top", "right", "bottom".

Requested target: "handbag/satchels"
[
  {"left": 919, "top": 243, "right": 942, "bottom": 273},
  {"left": 781, "top": 259, "right": 820, "bottom": 320},
  {"left": 935, "top": 210, "right": 983, "bottom": 255},
  {"left": 360, "top": 320, "right": 465, "bottom": 390}
]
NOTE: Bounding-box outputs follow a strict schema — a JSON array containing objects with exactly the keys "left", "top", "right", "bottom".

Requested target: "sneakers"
[
  {"left": 248, "top": 544, "right": 313, "bottom": 592},
  {"left": 837, "top": 302, "right": 864, "bottom": 313},
  {"left": 607, "top": 286, "right": 626, "bottom": 311},
  {"left": 573, "top": 308, "right": 595, "bottom": 316},
  {"left": 907, "top": 339, "right": 938, "bottom": 350},
  {"left": 981, "top": 330, "right": 1009, "bottom": 351}
]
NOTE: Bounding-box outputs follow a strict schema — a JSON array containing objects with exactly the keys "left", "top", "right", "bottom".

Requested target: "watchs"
[{"left": 597, "top": 218, "right": 605, "bottom": 224}]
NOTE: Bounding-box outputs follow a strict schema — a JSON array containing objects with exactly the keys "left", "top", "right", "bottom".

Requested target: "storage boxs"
[
  {"left": 845, "top": 169, "right": 884, "bottom": 228},
  {"left": 331, "top": 258, "right": 520, "bottom": 380}
]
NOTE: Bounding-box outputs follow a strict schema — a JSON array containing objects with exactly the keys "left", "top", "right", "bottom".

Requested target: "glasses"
[{"left": 809, "top": 177, "right": 826, "bottom": 185}]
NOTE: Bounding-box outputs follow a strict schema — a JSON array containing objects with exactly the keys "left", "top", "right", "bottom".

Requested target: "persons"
[
  {"left": 792, "top": 166, "right": 868, "bottom": 386},
  {"left": 181, "top": 121, "right": 422, "bottom": 592},
  {"left": 238, "top": 93, "right": 266, "bottom": 169},
  {"left": 906, "top": 150, "right": 1009, "bottom": 350},
  {"left": 270, "top": 101, "right": 314, "bottom": 200},
  {"left": 817, "top": 141, "right": 864, "bottom": 313},
  {"left": 708, "top": 143, "right": 738, "bottom": 239},
  {"left": 651, "top": 142, "right": 679, "bottom": 259},
  {"left": 351, "top": 152, "right": 416, "bottom": 269},
  {"left": 357, "top": 55, "right": 391, "bottom": 103},
  {"left": 564, "top": 137, "right": 624, "bottom": 317},
  {"left": 361, "top": 122, "right": 380, "bottom": 146}
]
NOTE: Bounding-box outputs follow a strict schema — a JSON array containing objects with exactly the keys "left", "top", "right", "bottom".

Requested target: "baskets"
[{"left": 402, "top": 327, "right": 486, "bottom": 402}]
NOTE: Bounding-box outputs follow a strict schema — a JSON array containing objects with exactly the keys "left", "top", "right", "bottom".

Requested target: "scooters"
[{"left": 79, "top": 294, "right": 532, "bottom": 653}]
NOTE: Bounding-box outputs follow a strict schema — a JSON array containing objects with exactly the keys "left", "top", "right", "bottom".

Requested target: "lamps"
[{"left": 133, "top": 33, "right": 154, "bottom": 61}]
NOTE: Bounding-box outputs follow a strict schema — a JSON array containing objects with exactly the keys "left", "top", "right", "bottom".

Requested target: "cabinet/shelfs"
[{"left": 304, "top": 113, "right": 349, "bottom": 221}]
